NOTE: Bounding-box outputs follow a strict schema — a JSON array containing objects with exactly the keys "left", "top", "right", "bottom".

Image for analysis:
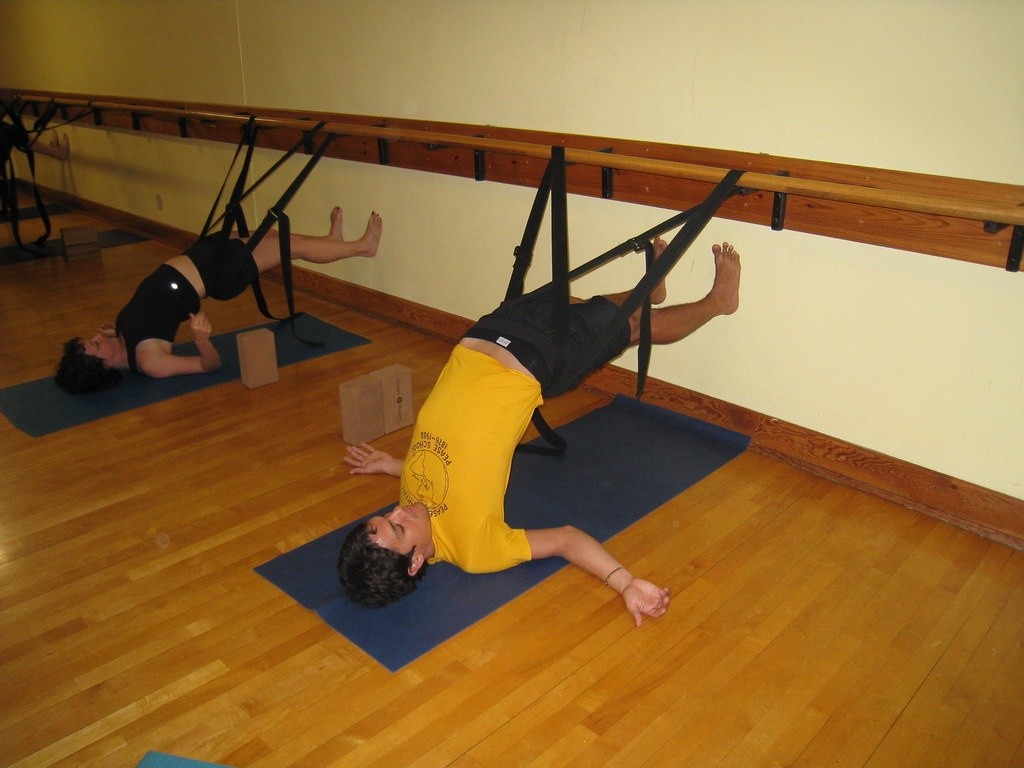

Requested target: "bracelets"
[{"left": 604, "top": 567, "right": 623, "bottom": 585}]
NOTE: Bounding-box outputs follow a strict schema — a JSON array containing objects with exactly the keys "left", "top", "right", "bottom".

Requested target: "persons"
[
  {"left": 338, "top": 237, "right": 741, "bottom": 628},
  {"left": 55, "top": 206, "right": 383, "bottom": 394},
  {"left": 0, "top": 121, "right": 69, "bottom": 164}
]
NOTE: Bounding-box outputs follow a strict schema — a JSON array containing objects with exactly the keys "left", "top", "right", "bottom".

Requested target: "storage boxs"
[
  {"left": 61, "top": 224, "right": 102, "bottom": 260},
  {"left": 235, "top": 328, "right": 279, "bottom": 389},
  {"left": 338, "top": 374, "right": 384, "bottom": 445},
  {"left": 370, "top": 365, "right": 414, "bottom": 434}
]
